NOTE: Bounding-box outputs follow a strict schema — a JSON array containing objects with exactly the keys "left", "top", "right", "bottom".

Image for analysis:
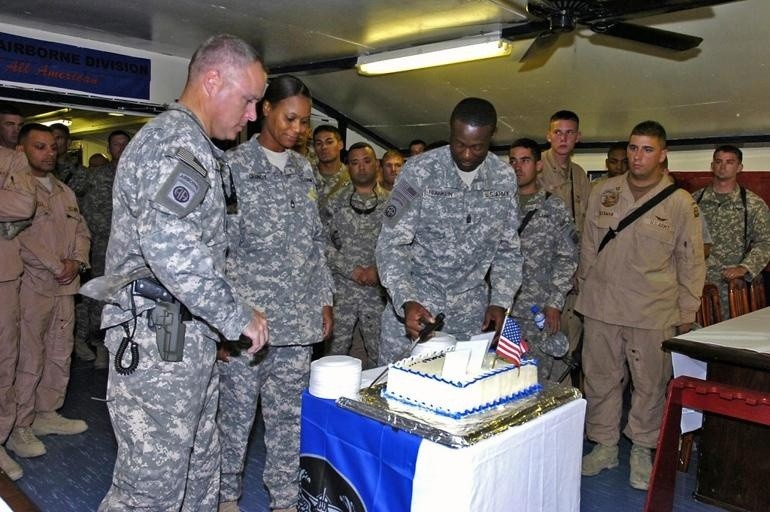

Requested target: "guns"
[{"left": 130, "top": 277, "right": 176, "bottom": 305}]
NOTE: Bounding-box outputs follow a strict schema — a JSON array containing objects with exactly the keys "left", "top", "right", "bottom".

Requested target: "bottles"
[{"left": 531, "top": 305, "right": 547, "bottom": 331}]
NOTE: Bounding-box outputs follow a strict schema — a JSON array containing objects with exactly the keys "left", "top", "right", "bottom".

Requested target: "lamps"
[{"left": 354, "top": 31, "right": 513, "bottom": 77}]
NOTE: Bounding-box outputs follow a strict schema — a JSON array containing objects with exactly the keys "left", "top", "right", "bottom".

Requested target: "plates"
[{"left": 308, "top": 354, "right": 363, "bottom": 401}]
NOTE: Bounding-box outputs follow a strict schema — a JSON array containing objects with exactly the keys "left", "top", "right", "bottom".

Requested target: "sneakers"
[
  {"left": 582, "top": 444, "right": 619, "bottom": 476},
  {"left": 31, "top": 411, "right": 89, "bottom": 435},
  {"left": 5, "top": 427, "right": 47, "bottom": 458},
  {"left": 74, "top": 342, "right": 96, "bottom": 361},
  {"left": 94, "top": 345, "right": 107, "bottom": 369},
  {"left": 630, "top": 444, "right": 653, "bottom": 490}
]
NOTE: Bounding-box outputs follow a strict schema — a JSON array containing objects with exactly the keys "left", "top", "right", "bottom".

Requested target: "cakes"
[{"left": 380, "top": 307, "right": 542, "bottom": 419}]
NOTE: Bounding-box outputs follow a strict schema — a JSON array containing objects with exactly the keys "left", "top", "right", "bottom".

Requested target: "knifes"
[{"left": 368, "top": 312, "right": 446, "bottom": 389}]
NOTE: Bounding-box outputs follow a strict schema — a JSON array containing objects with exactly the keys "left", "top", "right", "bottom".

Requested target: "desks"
[
  {"left": 298, "top": 360, "right": 587, "bottom": 512},
  {"left": 661, "top": 305, "right": 770, "bottom": 511}
]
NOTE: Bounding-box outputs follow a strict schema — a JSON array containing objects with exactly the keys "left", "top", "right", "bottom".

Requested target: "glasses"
[
  {"left": 219, "top": 165, "right": 238, "bottom": 214},
  {"left": 350, "top": 189, "right": 378, "bottom": 214}
]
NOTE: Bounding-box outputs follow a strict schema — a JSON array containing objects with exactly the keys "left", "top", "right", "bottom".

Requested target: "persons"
[
  {"left": 51, "top": 124, "right": 131, "bottom": 371},
  {"left": 0, "top": 110, "right": 90, "bottom": 480},
  {"left": 575, "top": 120, "right": 770, "bottom": 492},
  {"left": 79, "top": 33, "right": 269, "bottom": 512},
  {"left": 217, "top": 74, "right": 334, "bottom": 512},
  {"left": 298, "top": 96, "right": 592, "bottom": 381}
]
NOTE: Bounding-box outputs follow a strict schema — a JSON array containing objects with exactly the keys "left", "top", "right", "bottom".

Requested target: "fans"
[{"left": 489, "top": 0, "right": 743, "bottom": 63}]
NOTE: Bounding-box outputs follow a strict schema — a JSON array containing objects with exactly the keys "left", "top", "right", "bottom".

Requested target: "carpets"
[{"left": 16, "top": 359, "right": 740, "bottom": 512}]
